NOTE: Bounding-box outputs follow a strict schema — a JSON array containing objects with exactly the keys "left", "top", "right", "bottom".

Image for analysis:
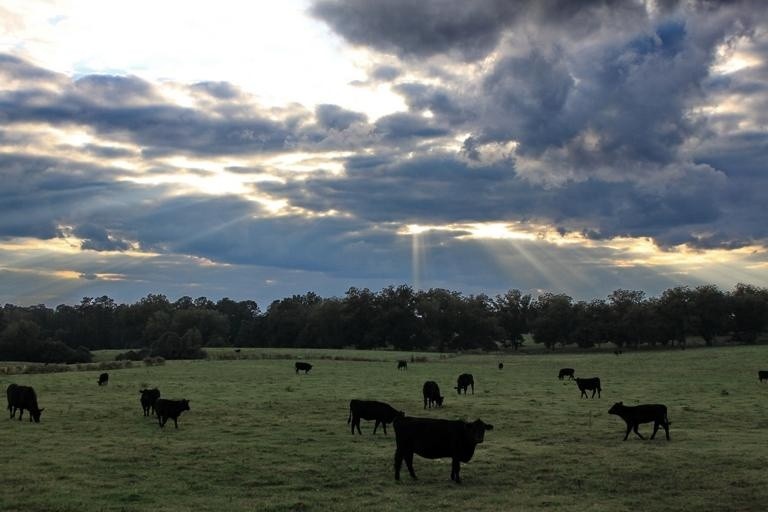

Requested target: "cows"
[
  {"left": 397, "top": 360, "right": 407, "bottom": 372},
  {"left": 423, "top": 380, "right": 444, "bottom": 410},
  {"left": 499, "top": 362, "right": 503, "bottom": 369},
  {"left": 574, "top": 377, "right": 602, "bottom": 400},
  {"left": 393, "top": 415, "right": 494, "bottom": 483},
  {"left": 155, "top": 398, "right": 191, "bottom": 429},
  {"left": 139, "top": 386, "right": 160, "bottom": 417},
  {"left": 347, "top": 399, "right": 405, "bottom": 436},
  {"left": 97, "top": 373, "right": 108, "bottom": 386},
  {"left": 454, "top": 373, "right": 474, "bottom": 396},
  {"left": 295, "top": 361, "right": 312, "bottom": 374},
  {"left": 758, "top": 371, "right": 768, "bottom": 382},
  {"left": 6, "top": 383, "right": 45, "bottom": 425},
  {"left": 608, "top": 401, "right": 671, "bottom": 441},
  {"left": 234, "top": 349, "right": 241, "bottom": 353},
  {"left": 557, "top": 368, "right": 574, "bottom": 381}
]
